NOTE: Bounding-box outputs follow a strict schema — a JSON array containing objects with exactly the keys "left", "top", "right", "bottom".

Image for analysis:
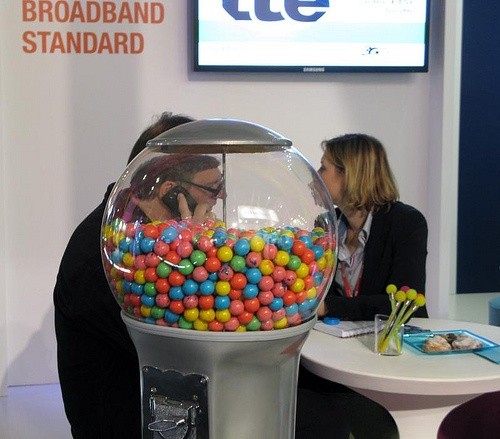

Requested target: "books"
[{"left": 312, "top": 320, "right": 400, "bottom": 338}]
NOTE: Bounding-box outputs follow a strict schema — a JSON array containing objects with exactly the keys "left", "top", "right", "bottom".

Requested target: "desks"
[{"left": 300, "top": 318, "right": 500, "bottom": 439}]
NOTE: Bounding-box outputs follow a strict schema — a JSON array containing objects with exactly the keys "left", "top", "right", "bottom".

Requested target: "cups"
[{"left": 375, "top": 315, "right": 404, "bottom": 357}]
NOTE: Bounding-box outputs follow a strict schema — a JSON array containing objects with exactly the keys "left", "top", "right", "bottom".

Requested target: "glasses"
[{"left": 174, "top": 172, "right": 225, "bottom": 198}]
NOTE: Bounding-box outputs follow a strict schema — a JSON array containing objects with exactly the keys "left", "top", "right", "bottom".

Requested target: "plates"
[{"left": 404, "top": 329, "right": 499, "bottom": 356}]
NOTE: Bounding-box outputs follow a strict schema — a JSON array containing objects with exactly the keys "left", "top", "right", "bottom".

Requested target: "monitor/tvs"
[{"left": 194, "top": 0, "right": 431, "bottom": 71}]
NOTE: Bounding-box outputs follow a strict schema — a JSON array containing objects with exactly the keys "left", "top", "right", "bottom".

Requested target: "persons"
[
  {"left": 51, "top": 110, "right": 357, "bottom": 439},
  {"left": 311, "top": 132, "right": 428, "bottom": 318}
]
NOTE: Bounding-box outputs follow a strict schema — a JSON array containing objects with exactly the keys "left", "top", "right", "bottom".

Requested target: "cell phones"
[{"left": 161, "top": 186, "right": 198, "bottom": 217}]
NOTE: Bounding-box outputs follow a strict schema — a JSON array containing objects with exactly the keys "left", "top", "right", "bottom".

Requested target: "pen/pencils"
[{"left": 376, "top": 284, "right": 426, "bottom": 354}]
